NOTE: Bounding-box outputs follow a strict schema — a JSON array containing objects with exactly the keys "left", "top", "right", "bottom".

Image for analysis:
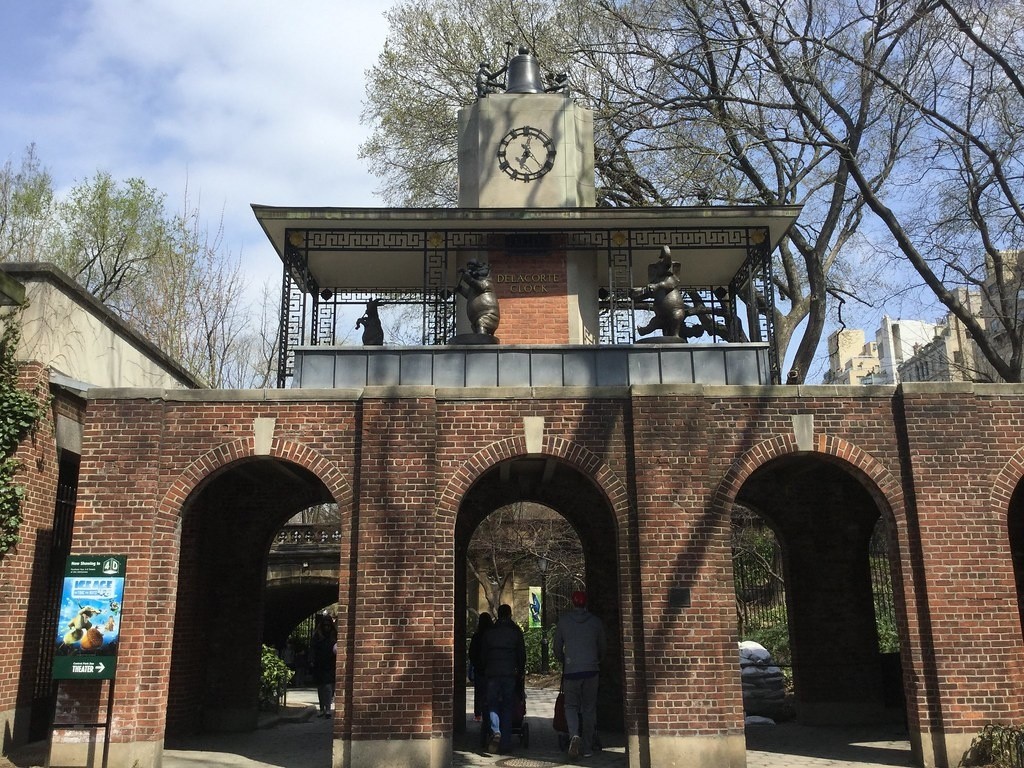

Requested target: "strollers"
[
  {"left": 553, "top": 665, "right": 602, "bottom": 751},
  {"left": 478, "top": 668, "right": 529, "bottom": 749}
]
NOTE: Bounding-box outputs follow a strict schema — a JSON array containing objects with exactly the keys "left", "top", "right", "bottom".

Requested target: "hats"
[{"left": 572, "top": 591, "right": 587, "bottom": 607}]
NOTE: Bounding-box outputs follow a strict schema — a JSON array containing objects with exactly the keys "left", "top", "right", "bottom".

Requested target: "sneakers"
[{"left": 472, "top": 715, "right": 483, "bottom": 722}]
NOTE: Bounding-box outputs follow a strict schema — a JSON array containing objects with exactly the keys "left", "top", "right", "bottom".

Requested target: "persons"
[
  {"left": 553, "top": 592, "right": 608, "bottom": 760},
  {"left": 469, "top": 612, "right": 495, "bottom": 722},
  {"left": 311, "top": 615, "right": 337, "bottom": 719},
  {"left": 479, "top": 604, "right": 525, "bottom": 755},
  {"left": 476, "top": 61, "right": 508, "bottom": 95}
]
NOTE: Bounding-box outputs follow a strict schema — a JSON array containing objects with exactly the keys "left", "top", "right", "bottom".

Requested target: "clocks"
[{"left": 497, "top": 126, "right": 557, "bottom": 183}]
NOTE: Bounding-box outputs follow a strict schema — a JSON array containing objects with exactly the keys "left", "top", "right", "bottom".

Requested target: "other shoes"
[
  {"left": 567, "top": 735, "right": 580, "bottom": 764},
  {"left": 583, "top": 750, "right": 595, "bottom": 758},
  {"left": 487, "top": 731, "right": 500, "bottom": 755},
  {"left": 317, "top": 712, "right": 325, "bottom": 718},
  {"left": 325, "top": 712, "right": 332, "bottom": 718}
]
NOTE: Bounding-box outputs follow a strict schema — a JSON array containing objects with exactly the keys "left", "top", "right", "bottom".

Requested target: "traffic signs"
[{"left": 51, "top": 654, "right": 115, "bottom": 680}]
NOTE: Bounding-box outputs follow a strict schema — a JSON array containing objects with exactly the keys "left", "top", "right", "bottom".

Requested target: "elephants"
[{"left": 628, "top": 245, "right": 685, "bottom": 337}]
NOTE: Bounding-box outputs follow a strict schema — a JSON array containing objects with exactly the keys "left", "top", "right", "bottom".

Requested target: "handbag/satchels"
[
  {"left": 468, "top": 666, "right": 476, "bottom": 683},
  {"left": 552, "top": 694, "right": 570, "bottom": 733}
]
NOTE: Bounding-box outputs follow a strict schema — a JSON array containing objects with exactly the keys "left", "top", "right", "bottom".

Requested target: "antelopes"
[{"left": 354, "top": 297, "right": 386, "bottom": 346}]
